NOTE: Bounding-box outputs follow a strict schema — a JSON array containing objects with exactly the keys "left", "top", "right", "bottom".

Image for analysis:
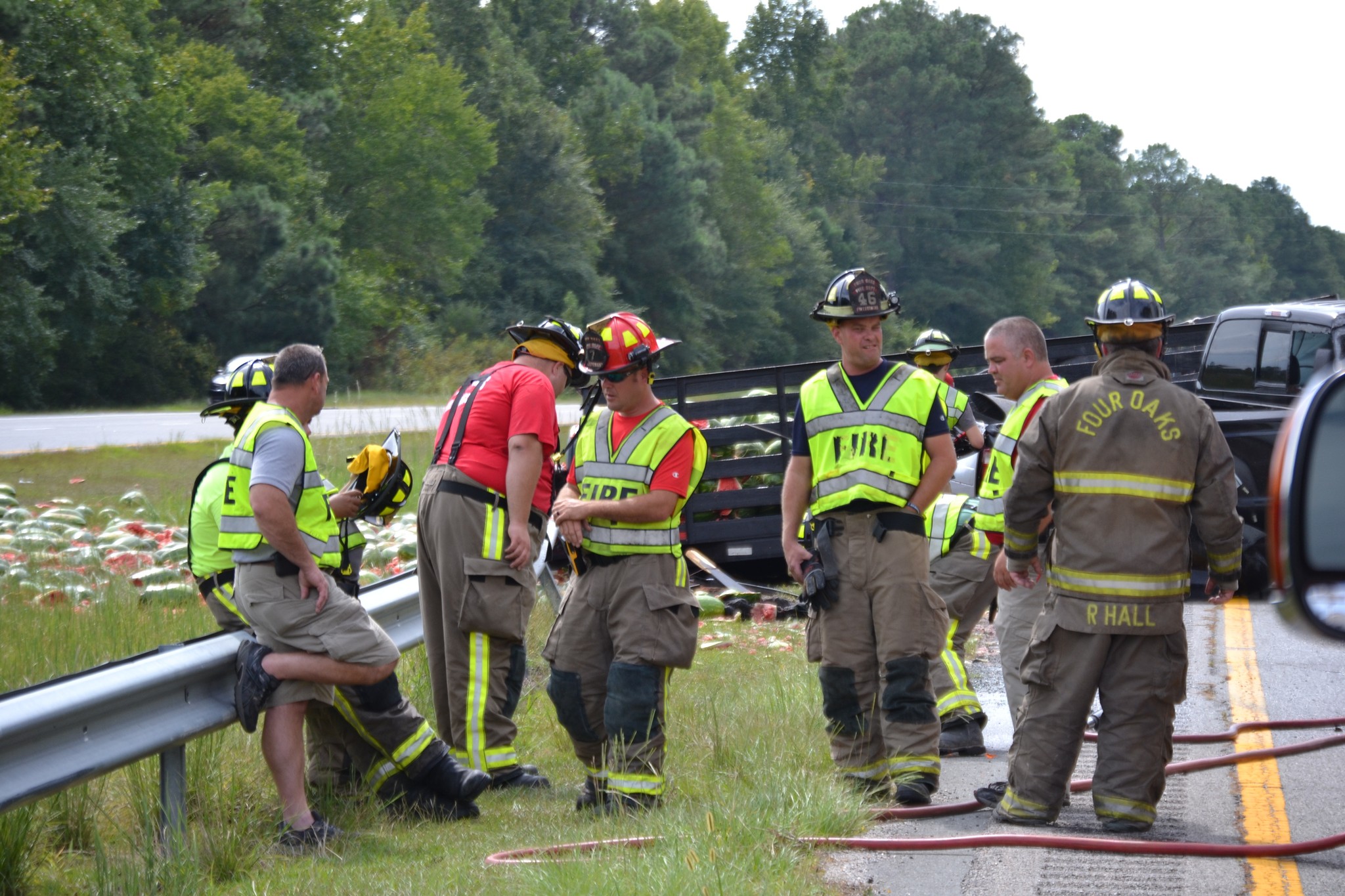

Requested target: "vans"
[{"left": 1195, "top": 302, "right": 1345, "bottom": 411}]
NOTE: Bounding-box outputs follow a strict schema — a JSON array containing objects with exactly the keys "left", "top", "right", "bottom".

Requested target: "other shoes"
[
  {"left": 938, "top": 718, "right": 986, "bottom": 757},
  {"left": 576, "top": 785, "right": 648, "bottom": 822},
  {"left": 376, "top": 772, "right": 479, "bottom": 825},
  {"left": 492, "top": 772, "right": 551, "bottom": 791},
  {"left": 424, "top": 752, "right": 492, "bottom": 805},
  {"left": 989, "top": 781, "right": 1071, "bottom": 807},
  {"left": 895, "top": 781, "right": 932, "bottom": 805},
  {"left": 843, "top": 776, "right": 891, "bottom": 802},
  {"left": 520, "top": 764, "right": 539, "bottom": 776},
  {"left": 974, "top": 788, "right": 1005, "bottom": 809}
]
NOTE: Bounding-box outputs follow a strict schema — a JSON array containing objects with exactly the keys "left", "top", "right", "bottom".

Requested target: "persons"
[
  {"left": 188, "top": 311, "right": 706, "bottom": 823},
  {"left": 990, "top": 280, "right": 1243, "bottom": 832},
  {"left": 907, "top": 315, "right": 1069, "bottom": 754},
  {"left": 780, "top": 267, "right": 958, "bottom": 807},
  {"left": 216, "top": 341, "right": 400, "bottom": 848}
]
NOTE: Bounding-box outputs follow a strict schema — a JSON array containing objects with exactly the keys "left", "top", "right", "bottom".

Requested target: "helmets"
[
  {"left": 505, "top": 315, "right": 591, "bottom": 388},
  {"left": 345, "top": 427, "right": 413, "bottom": 517},
  {"left": 200, "top": 358, "right": 275, "bottom": 418},
  {"left": 906, "top": 329, "right": 961, "bottom": 366},
  {"left": 578, "top": 312, "right": 682, "bottom": 375},
  {"left": 1084, "top": 278, "right": 1177, "bottom": 343},
  {"left": 809, "top": 267, "right": 901, "bottom": 323}
]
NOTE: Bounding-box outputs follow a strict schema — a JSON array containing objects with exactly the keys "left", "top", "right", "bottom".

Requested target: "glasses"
[{"left": 597, "top": 364, "right": 645, "bottom": 383}]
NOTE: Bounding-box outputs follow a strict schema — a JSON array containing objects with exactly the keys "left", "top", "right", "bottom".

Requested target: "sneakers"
[
  {"left": 273, "top": 810, "right": 355, "bottom": 851},
  {"left": 234, "top": 639, "right": 280, "bottom": 733}
]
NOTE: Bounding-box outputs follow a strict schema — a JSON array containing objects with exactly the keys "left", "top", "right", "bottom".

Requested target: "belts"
[
  {"left": 436, "top": 479, "right": 553, "bottom": 562},
  {"left": 199, "top": 568, "right": 235, "bottom": 601}
]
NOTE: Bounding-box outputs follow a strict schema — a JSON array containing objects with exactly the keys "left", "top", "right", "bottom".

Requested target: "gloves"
[{"left": 798, "top": 550, "right": 839, "bottom": 612}]
